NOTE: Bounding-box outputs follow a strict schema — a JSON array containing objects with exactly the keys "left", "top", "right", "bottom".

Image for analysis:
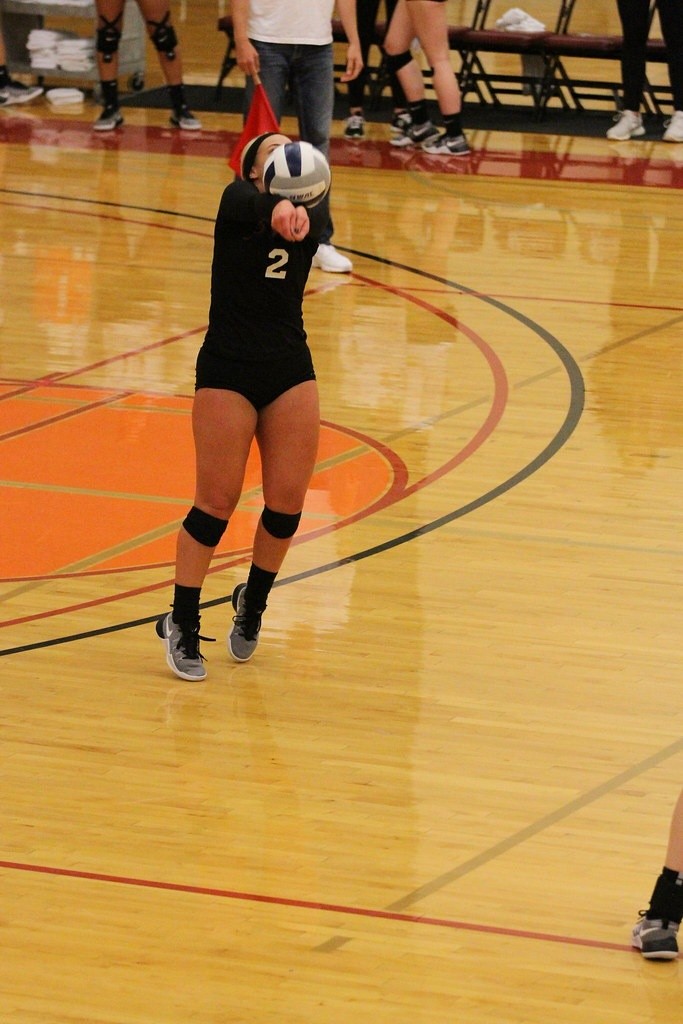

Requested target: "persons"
[
  {"left": 232, "top": 0, "right": 364, "bottom": 272},
  {"left": 630, "top": 790, "right": 683, "bottom": 960},
  {"left": 383, "top": 0, "right": 473, "bottom": 156},
  {"left": 606, "top": 0, "right": 683, "bottom": 141},
  {"left": 153, "top": 136, "right": 335, "bottom": 683},
  {"left": 94, "top": 0, "right": 203, "bottom": 131},
  {"left": 0, "top": 26, "right": 45, "bottom": 106},
  {"left": 343, "top": 0, "right": 414, "bottom": 137}
]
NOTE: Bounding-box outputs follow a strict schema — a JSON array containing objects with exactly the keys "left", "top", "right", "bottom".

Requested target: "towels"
[
  {"left": 25, "top": 27, "right": 96, "bottom": 72},
  {"left": 46, "top": 103, "right": 84, "bottom": 115},
  {"left": 493, "top": 7, "right": 555, "bottom": 96},
  {"left": 46, "top": 86, "right": 84, "bottom": 106}
]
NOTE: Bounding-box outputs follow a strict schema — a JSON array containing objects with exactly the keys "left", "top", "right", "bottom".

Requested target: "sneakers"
[
  {"left": 343, "top": 112, "right": 365, "bottom": 140},
  {"left": 607, "top": 109, "right": 646, "bottom": 141},
  {"left": 312, "top": 243, "right": 355, "bottom": 275},
  {"left": 391, "top": 112, "right": 414, "bottom": 134},
  {"left": 388, "top": 121, "right": 442, "bottom": 148},
  {"left": 227, "top": 583, "right": 267, "bottom": 663},
  {"left": 659, "top": 111, "right": 683, "bottom": 142},
  {"left": 632, "top": 909, "right": 680, "bottom": 962},
  {"left": 420, "top": 132, "right": 472, "bottom": 157},
  {"left": 156, "top": 611, "right": 217, "bottom": 682}
]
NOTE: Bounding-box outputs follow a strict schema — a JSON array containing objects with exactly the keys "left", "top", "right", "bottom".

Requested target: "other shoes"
[
  {"left": 168, "top": 110, "right": 203, "bottom": 130},
  {"left": 0, "top": 82, "right": 45, "bottom": 107},
  {"left": 91, "top": 108, "right": 124, "bottom": 132}
]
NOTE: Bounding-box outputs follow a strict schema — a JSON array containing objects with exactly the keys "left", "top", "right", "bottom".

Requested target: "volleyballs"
[{"left": 261, "top": 140, "right": 332, "bottom": 209}]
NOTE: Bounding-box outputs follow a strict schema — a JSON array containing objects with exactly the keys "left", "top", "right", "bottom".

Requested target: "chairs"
[{"left": 212, "top": 0, "right": 683, "bottom": 142}]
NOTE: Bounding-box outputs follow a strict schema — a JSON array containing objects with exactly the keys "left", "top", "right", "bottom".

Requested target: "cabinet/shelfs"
[{"left": 0, "top": 0, "right": 146, "bottom": 107}]
